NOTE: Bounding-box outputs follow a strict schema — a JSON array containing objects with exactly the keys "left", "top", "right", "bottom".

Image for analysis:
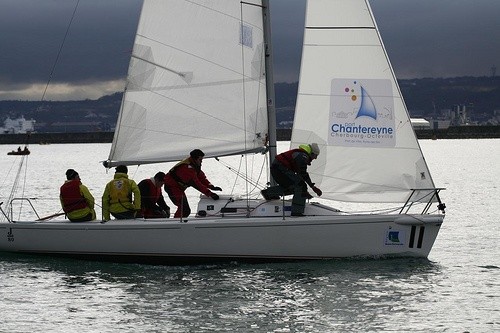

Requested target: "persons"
[
  {"left": 60, "top": 169, "right": 96, "bottom": 221},
  {"left": 137, "top": 171, "right": 171, "bottom": 218},
  {"left": 101, "top": 165, "right": 142, "bottom": 221},
  {"left": 260, "top": 142, "right": 323, "bottom": 217},
  {"left": 162, "top": 148, "right": 222, "bottom": 218},
  {"left": 17, "top": 146, "right": 30, "bottom": 152}
]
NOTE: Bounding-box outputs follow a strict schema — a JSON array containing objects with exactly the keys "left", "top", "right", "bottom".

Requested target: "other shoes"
[
  {"left": 291, "top": 212, "right": 306, "bottom": 218},
  {"left": 260, "top": 190, "right": 272, "bottom": 201}
]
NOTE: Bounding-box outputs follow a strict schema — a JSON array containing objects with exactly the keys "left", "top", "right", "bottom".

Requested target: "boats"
[{"left": 7, "top": 148, "right": 32, "bottom": 157}]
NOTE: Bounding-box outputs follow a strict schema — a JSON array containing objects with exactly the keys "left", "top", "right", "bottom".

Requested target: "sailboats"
[{"left": 0, "top": 0, "right": 447, "bottom": 259}]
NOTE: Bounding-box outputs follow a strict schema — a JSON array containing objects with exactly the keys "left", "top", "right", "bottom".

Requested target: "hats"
[
  {"left": 307, "top": 142, "right": 320, "bottom": 155},
  {"left": 65, "top": 169, "right": 78, "bottom": 180},
  {"left": 115, "top": 165, "right": 128, "bottom": 173},
  {"left": 190, "top": 149, "right": 205, "bottom": 157}
]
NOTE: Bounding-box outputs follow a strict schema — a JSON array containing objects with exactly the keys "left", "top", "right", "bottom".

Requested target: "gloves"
[
  {"left": 211, "top": 193, "right": 220, "bottom": 200},
  {"left": 313, "top": 186, "right": 322, "bottom": 197},
  {"left": 213, "top": 187, "right": 222, "bottom": 191}
]
